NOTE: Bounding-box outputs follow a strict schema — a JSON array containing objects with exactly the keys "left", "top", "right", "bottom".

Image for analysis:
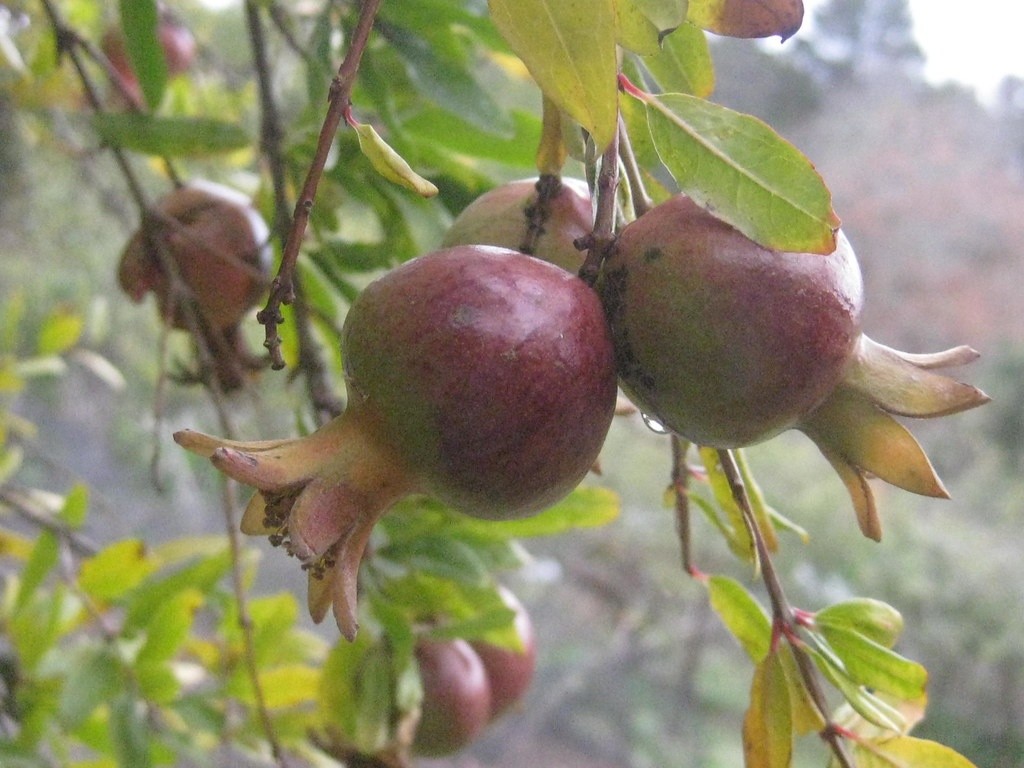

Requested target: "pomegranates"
[{"left": 80, "top": 0, "right": 989, "bottom": 768}]
